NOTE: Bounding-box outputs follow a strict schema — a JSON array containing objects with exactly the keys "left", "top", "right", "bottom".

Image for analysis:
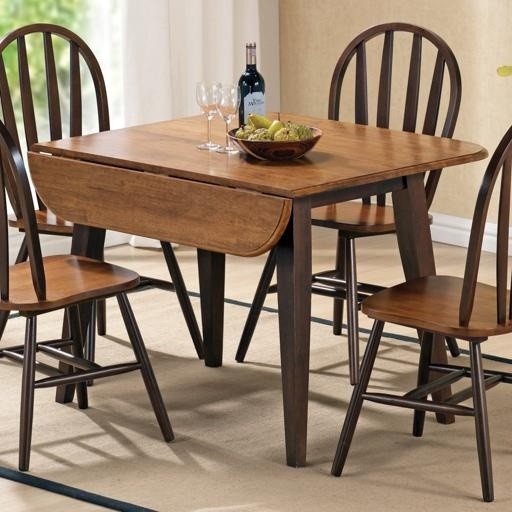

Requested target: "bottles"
[{"left": 238, "top": 43, "right": 266, "bottom": 158}]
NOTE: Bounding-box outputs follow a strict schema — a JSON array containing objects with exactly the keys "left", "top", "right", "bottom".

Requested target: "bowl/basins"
[{"left": 227, "top": 126, "right": 323, "bottom": 161}]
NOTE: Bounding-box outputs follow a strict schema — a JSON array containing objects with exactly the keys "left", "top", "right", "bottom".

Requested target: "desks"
[{"left": 28, "top": 108, "right": 489, "bottom": 467}]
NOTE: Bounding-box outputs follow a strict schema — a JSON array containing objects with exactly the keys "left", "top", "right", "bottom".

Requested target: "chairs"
[
  {"left": 0, "top": 23, "right": 205, "bottom": 387},
  {"left": 332, "top": 126, "right": 512, "bottom": 503},
  {"left": 235, "top": 23, "right": 463, "bottom": 386},
  {"left": 1, "top": 120, "right": 174, "bottom": 470}
]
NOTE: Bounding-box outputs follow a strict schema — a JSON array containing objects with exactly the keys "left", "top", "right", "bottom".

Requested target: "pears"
[
  {"left": 268, "top": 120, "right": 284, "bottom": 136},
  {"left": 247, "top": 113, "right": 272, "bottom": 128}
]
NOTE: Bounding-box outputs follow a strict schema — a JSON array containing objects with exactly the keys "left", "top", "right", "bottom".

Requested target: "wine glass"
[
  {"left": 195, "top": 80, "right": 221, "bottom": 151},
  {"left": 214, "top": 84, "right": 242, "bottom": 154}
]
